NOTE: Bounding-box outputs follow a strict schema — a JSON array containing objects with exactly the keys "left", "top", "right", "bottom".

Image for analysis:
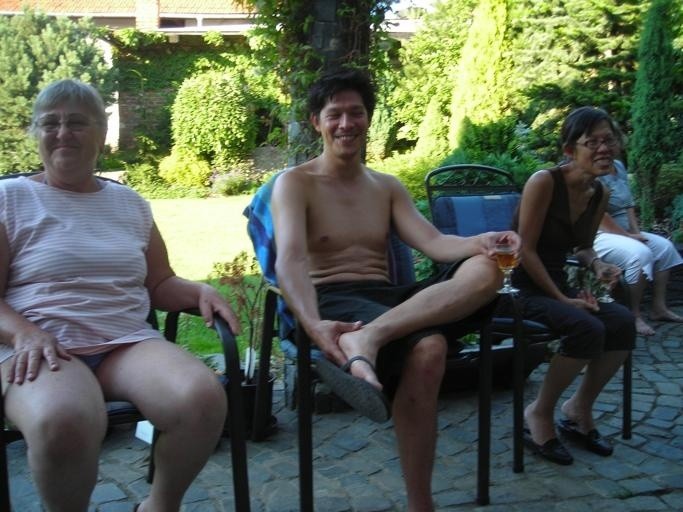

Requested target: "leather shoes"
[
  {"left": 557, "top": 414, "right": 614, "bottom": 457},
  {"left": 521, "top": 428, "right": 574, "bottom": 466}
]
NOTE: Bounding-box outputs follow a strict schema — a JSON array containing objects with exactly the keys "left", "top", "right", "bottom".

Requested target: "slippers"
[{"left": 316, "top": 353, "right": 390, "bottom": 425}]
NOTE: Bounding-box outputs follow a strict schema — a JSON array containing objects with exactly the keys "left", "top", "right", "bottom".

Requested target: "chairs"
[
  {"left": 256, "top": 162, "right": 499, "bottom": 505},
  {"left": 421, "top": 162, "right": 632, "bottom": 477},
  {"left": 0, "top": 172, "right": 251, "bottom": 512}
]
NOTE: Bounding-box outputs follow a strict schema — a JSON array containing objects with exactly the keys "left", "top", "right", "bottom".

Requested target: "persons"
[
  {"left": 0, "top": 79, "right": 241, "bottom": 512},
  {"left": 272, "top": 71, "right": 522, "bottom": 512},
  {"left": 507, "top": 107, "right": 637, "bottom": 465},
  {"left": 592, "top": 120, "right": 681, "bottom": 336}
]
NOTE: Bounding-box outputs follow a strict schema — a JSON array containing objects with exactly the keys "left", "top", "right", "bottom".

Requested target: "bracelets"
[{"left": 589, "top": 256, "right": 600, "bottom": 272}]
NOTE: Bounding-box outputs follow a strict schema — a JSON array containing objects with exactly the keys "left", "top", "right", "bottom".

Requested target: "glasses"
[{"left": 571, "top": 136, "right": 619, "bottom": 148}]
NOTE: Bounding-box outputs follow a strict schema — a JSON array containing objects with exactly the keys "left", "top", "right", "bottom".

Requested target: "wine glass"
[
  {"left": 496, "top": 241, "right": 519, "bottom": 295},
  {"left": 597, "top": 267, "right": 615, "bottom": 302}
]
